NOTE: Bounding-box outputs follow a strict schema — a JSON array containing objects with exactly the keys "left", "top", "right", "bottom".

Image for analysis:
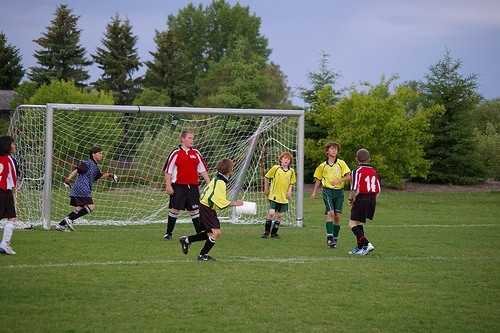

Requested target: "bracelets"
[{"left": 349, "top": 197, "right": 353, "bottom": 201}]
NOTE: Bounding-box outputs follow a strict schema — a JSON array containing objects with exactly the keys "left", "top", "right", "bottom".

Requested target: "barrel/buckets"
[{"left": 236, "top": 200, "right": 257, "bottom": 216}]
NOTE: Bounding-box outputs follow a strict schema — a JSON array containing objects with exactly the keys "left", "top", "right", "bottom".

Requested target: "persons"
[
  {"left": 311, "top": 141, "right": 351, "bottom": 249},
  {"left": 0, "top": 135, "right": 17, "bottom": 254},
  {"left": 55, "top": 147, "right": 117, "bottom": 232},
  {"left": 261, "top": 152, "right": 296, "bottom": 238},
  {"left": 180, "top": 158, "right": 243, "bottom": 261},
  {"left": 348, "top": 148, "right": 381, "bottom": 255},
  {"left": 161, "top": 131, "right": 210, "bottom": 241}
]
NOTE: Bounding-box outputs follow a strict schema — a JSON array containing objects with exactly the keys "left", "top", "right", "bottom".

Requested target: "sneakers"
[
  {"left": 55, "top": 223, "right": 70, "bottom": 232},
  {"left": 0, "top": 243, "right": 17, "bottom": 255},
  {"left": 163, "top": 234, "right": 172, "bottom": 241},
  {"left": 361, "top": 243, "right": 375, "bottom": 255},
  {"left": 64, "top": 216, "right": 75, "bottom": 231},
  {"left": 348, "top": 247, "right": 363, "bottom": 254},
  {"left": 331, "top": 237, "right": 338, "bottom": 247},
  {"left": 197, "top": 254, "right": 216, "bottom": 261},
  {"left": 327, "top": 236, "right": 336, "bottom": 245},
  {"left": 271, "top": 233, "right": 280, "bottom": 238},
  {"left": 262, "top": 231, "right": 269, "bottom": 238},
  {"left": 180, "top": 236, "right": 189, "bottom": 255}
]
